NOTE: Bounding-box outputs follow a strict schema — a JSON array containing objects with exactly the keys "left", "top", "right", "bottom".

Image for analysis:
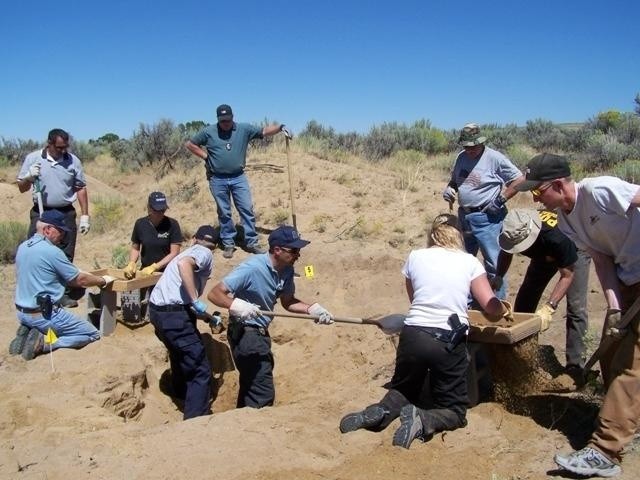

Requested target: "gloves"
[
  {"left": 140, "top": 263, "right": 162, "bottom": 278},
  {"left": 500, "top": 299, "right": 515, "bottom": 323},
  {"left": 279, "top": 124, "right": 294, "bottom": 140},
  {"left": 191, "top": 300, "right": 207, "bottom": 313},
  {"left": 487, "top": 194, "right": 507, "bottom": 224},
  {"left": 228, "top": 298, "right": 262, "bottom": 322},
  {"left": 212, "top": 314, "right": 223, "bottom": 325},
  {"left": 22, "top": 161, "right": 42, "bottom": 186},
  {"left": 122, "top": 260, "right": 137, "bottom": 280},
  {"left": 605, "top": 307, "right": 628, "bottom": 342},
  {"left": 100, "top": 274, "right": 117, "bottom": 291},
  {"left": 443, "top": 184, "right": 457, "bottom": 204},
  {"left": 78, "top": 214, "right": 92, "bottom": 236},
  {"left": 534, "top": 302, "right": 554, "bottom": 334},
  {"left": 306, "top": 302, "right": 335, "bottom": 326}
]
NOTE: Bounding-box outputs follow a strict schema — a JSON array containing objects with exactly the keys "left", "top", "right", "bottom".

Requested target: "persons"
[
  {"left": 148, "top": 224, "right": 222, "bottom": 421},
  {"left": 9, "top": 209, "right": 118, "bottom": 361},
  {"left": 443, "top": 123, "right": 524, "bottom": 310},
  {"left": 17, "top": 129, "right": 90, "bottom": 308},
  {"left": 340, "top": 214, "right": 514, "bottom": 450},
  {"left": 206, "top": 225, "right": 333, "bottom": 409},
  {"left": 123, "top": 192, "right": 183, "bottom": 280},
  {"left": 490, "top": 207, "right": 591, "bottom": 392},
  {"left": 185, "top": 104, "right": 292, "bottom": 258},
  {"left": 513, "top": 151, "right": 640, "bottom": 478}
]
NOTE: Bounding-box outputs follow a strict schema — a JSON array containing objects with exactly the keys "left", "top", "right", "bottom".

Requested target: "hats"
[
  {"left": 457, "top": 122, "right": 488, "bottom": 148},
  {"left": 215, "top": 103, "right": 234, "bottom": 123},
  {"left": 267, "top": 225, "right": 311, "bottom": 251},
  {"left": 146, "top": 191, "right": 171, "bottom": 212},
  {"left": 496, "top": 206, "right": 544, "bottom": 255},
  {"left": 193, "top": 225, "right": 221, "bottom": 244},
  {"left": 38, "top": 208, "right": 72, "bottom": 234},
  {"left": 511, "top": 151, "right": 572, "bottom": 193},
  {"left": 432, "top": 212, "right": 463, "bottom": 234}
]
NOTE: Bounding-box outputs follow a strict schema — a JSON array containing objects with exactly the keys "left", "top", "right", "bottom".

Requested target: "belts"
[
  {"left": 461, "top": 201, "right": 490, "bottom": 214},
  {"left": 147, "top": 301, "right": 191, "bottom": 313},
  {"left": 419, "top": 326, "right": 451, "bottom": 343},
  {"left": 14, "top": 302, "right": 41, "bottom": 314}
]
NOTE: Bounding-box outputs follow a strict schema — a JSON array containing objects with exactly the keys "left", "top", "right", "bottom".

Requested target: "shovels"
[
  {"left": 204, "top": 312, "right": 228, "bottom": 334},
  {"left": 261, "top": 311, "right": 407, "bottom": 334},
  {"left": 543, "top": 295, "right": 640, "bottom": 392}
]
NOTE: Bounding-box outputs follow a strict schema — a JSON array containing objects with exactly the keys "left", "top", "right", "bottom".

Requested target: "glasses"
[
  {"left": 531, "top": 184, "right": 552, "bottom": 197},
  {"left": 225, "top": 134, "right": 231, "bottom": 150}
]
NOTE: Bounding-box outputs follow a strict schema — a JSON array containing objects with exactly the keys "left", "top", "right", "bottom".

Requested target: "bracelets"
[{"left": 547, "top": 300, "right": 557, "bottom": 310}]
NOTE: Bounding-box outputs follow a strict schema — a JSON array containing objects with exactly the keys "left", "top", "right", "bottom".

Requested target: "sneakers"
[
  {"left": 552, "top": 444, "right": 623, "bottom": 477},
  {"left": 392, "top": 402, "right": 424, "bottom": 450},
  {"left": 244, "top": 243, "right": 267, "bottom": 254},
  {"left": 221, "top": 246, "right": 235, "bottom": 258},
  {"left": 7, "top": 324, "right": 29, "bottom": 355},
  {"left": 60, "top": 294, "right": 79, "bottom": 308},
  {"left": 339, "top": 403, "right": 389, "bottom": 435},
  {"left": 21, "top": 328, "right": 45, "bottom": 362}
]
